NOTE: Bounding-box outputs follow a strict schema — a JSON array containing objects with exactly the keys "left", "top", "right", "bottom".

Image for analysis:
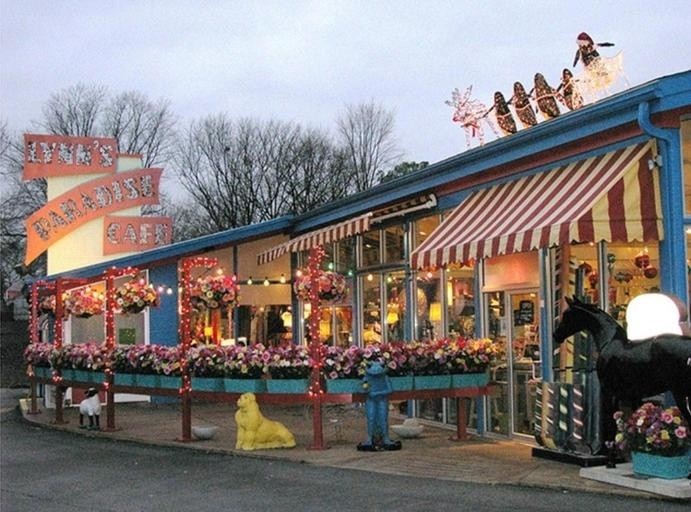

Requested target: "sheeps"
[{"left": 79, "top": 387, "right": 102, "bottom": 427}]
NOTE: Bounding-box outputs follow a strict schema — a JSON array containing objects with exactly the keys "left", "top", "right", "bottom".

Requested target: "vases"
[
  {"left": 319, "top": 288, "right": 340, "bottom": 301},
  {"left": 78, "top": 312, "right": 93, "bottom": 319},
  {"left": 123, "top": 302, "right": 147, "bottom": 313},
  {"left": 198, "top": 293, "right": 228, "bottom": 310}
]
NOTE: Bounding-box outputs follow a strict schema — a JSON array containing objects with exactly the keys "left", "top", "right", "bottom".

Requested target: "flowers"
[
  {"left": 113, "top": 279, "right": 161, "bottom": 314},
  {"left": 39, "top": 295, "right": 58, "bottom": 318},
  {"left": 63, "top": 286, "right": 106, "bottom": 320},
  {"left": 614, "top": 400, "right": 691, "bottom": 456},
  {"left": 293, "top": 270, "right": 348, "bottom": 304},
  {"left": 191, "top": 274, "right": 241, "bottom": 312}
]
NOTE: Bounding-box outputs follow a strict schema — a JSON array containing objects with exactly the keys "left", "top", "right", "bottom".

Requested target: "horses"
[{"left": 553, "top": 295, "right": 691, "bottom": 469}]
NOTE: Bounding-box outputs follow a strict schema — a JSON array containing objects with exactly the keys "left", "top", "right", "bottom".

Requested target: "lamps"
[
  {"left": 247, "top": 273, "right": 288, "bottom": 287},
  {"left": 149, "top": 281, "right": 174, "bottom": 296}
]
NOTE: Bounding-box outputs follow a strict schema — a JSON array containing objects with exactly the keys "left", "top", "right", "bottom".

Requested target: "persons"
[{"left": 267, "top": 306, "right": 288, "bottom": 347}]
